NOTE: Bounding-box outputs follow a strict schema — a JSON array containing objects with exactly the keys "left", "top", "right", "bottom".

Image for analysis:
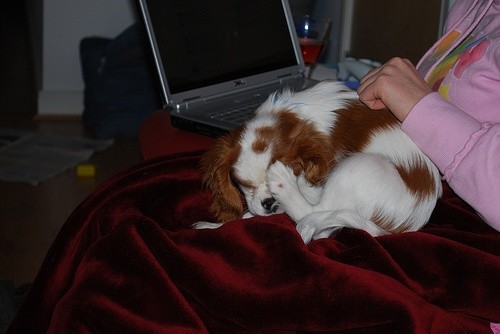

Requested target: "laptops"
[{"left": 138, "top": 0, "right": 324, "bottom": 140}]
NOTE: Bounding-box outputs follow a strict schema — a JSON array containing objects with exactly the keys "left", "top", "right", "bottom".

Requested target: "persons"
[{"left": 88, "top": 0, "right": 500, "bottom": 334}]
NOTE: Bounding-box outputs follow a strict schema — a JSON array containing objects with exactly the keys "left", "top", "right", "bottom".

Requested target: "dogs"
[{"left": 189, "top": 79, "right": 442, "bottom": 246}]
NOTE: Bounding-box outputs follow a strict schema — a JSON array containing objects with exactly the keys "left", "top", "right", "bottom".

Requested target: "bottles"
[{"left": 296, "top": 11, "right": 321, "bottom": 67}]
